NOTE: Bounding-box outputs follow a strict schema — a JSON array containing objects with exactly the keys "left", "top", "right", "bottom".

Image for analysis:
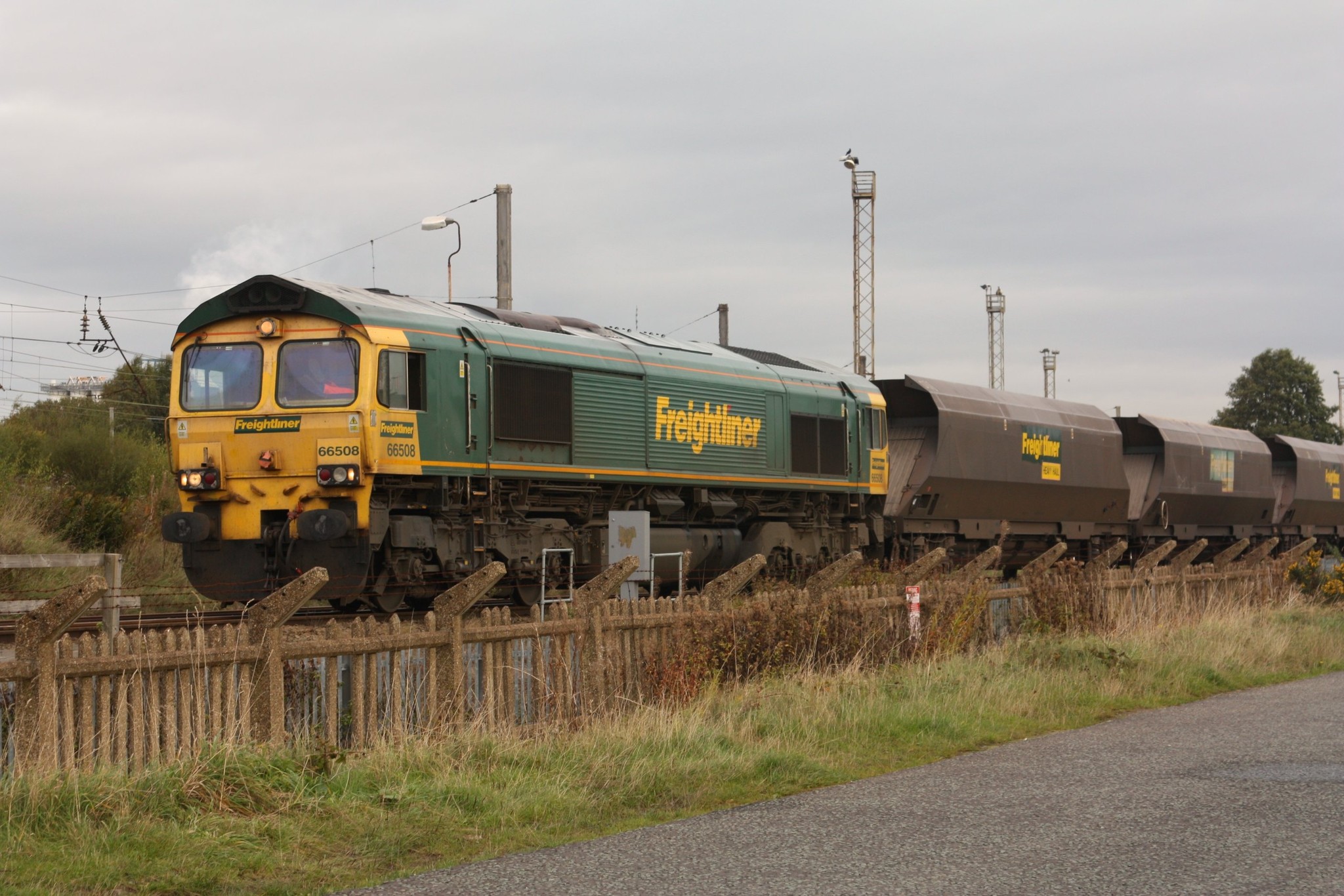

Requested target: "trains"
[{"left": 159, "top": 274, "right": 1344, "bottom": 631}]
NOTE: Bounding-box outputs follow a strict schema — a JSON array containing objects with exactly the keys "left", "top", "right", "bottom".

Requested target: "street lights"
[{"left": 421, "top": 215, "right": 461, "bottom": 303}]
